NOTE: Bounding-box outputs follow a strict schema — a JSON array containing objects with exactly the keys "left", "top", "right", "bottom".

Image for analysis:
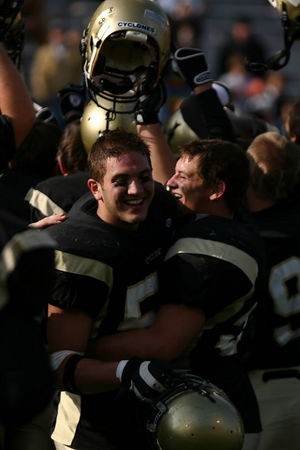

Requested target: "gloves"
[{"left": 116, "top": 356, "right": 173, "bottom": 404}]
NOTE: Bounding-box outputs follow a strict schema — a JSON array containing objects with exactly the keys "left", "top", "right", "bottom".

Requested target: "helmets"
[
  {"left": 167, "top": 88, "right": 232, "bottom": 153},
  {"left": 267, "top": 0, "right": 300, "bottom": 40},
  {"left": 80, "top": 0, "right": 171, "bottom": 91},
  {"left": 80, "top": 88, "right": 141, "bottom": 155},
  {"left": 149, "top": 374, "right": 245, "bottom": 450}
]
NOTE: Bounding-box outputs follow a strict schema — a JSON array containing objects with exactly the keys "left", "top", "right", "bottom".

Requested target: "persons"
[
  {"left": 34, "top": 126, "right": 243, "bottom": 448},
  {"left": 39, "top": 138, "right": 260, "bottom": 448},
  {"left": 2, "top": 0, "right": 299, "bottom": 230},
  {"left": 1, "top": 114, "right": 69, "bottom": 277},
  {"left": 208, "top": 129, "right": 300, "bottom": 450}
]
